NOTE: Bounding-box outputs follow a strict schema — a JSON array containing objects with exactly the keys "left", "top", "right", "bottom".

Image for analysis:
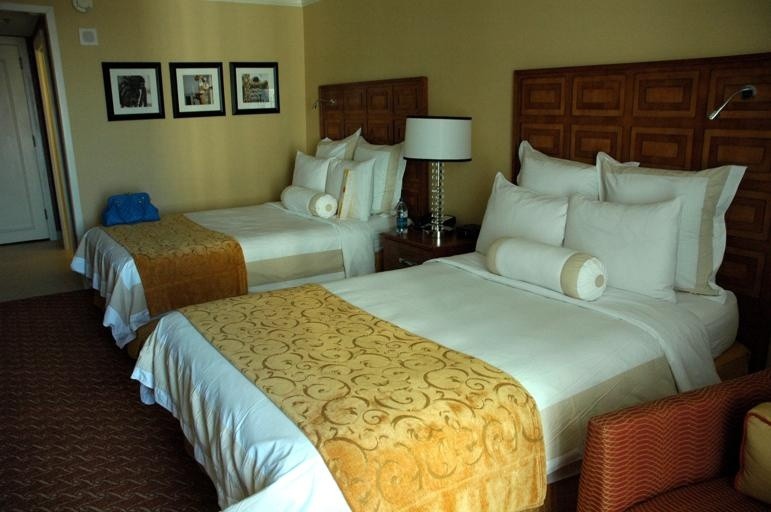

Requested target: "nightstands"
[{"left": 380, "top": 221, "right": 479, "bottom": 272}]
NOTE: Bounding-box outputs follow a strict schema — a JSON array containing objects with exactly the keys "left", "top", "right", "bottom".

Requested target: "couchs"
[{"left": 579, "top": 363, "right": 771, "bottom": 512}]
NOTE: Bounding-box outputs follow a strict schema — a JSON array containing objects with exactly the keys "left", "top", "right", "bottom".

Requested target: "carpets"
[{"left": 1, "top": 285, "right": 221, "bottom": 512}]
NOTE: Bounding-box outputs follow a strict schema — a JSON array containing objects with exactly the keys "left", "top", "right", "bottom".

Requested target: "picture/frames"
[
  {"left": 101, "top": 61, "right": 166, "bottom": 121},
  {"left": 168, "top": 61, "right": 226, "bottom": 120},
  {"left": 229, "top": 61, "right": 281, "bottom": 116}
]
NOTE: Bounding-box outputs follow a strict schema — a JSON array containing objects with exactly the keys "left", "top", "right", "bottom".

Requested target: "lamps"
[
  {"left": 709, "top": 85, "right": 757, "bottom": 122},
  {"left": 312, "top": 98, "right": 337, "bottom": 111},
  {"left": 403, "top": 115, "right": 472, "bottom": 238}
]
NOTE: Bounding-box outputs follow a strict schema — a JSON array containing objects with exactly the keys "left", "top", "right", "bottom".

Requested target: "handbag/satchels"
[{"left": 102, "top": 193, "right": 161, "bottom": 227}]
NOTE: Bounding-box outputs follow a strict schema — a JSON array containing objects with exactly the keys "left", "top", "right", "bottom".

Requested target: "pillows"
[
  {"left": 281, "top": 185, "right": 338, "bottom": 218},
  {"left": 597, "top": 151, "right": 747, "bottom": 301},
  {"left": 514, "top": 141, "right": 640, "bottom": 200},
  {"left": 734, "top": 402, "right": 771, "bottom": 500},
  {"left": 316, "top": 126, "right": 362, "bottom": 160},
  {"left": 353, "top": 136, "right": 409, "bottom": 214},
  {"left": 475, "top": 171, "right": 569, "bottom": 257},
  {"left": 485, "top": 236, "right": 609, "bottom": 301},
  {"left": 325, "top": 158, "right": 376, "bottom": 223},
  {"left": 564, "top": 192, "right": 684, "bottom": 306},
  {"left": 292, "top": 151, "right": 335, "bottom": 194}
]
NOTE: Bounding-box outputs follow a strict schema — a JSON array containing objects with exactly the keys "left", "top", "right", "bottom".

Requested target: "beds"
[
  {"left": 130, "top": 253, "right": 749, "bottom": 512},
  {"left": 72, "top": 202, "right": 413, "bottom": 359}
]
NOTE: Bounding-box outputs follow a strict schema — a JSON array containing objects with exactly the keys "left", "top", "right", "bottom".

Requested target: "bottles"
[{"left": 395, "top": 198, "right": 409, "bottom": 233}]
